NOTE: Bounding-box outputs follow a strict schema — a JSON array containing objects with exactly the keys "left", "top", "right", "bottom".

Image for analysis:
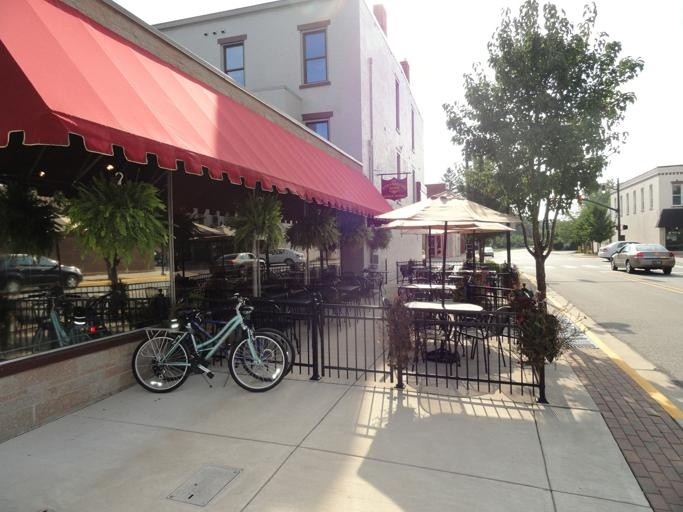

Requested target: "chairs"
[
  {"left": 382, "top": 263, "right": 513, "bottom": 379},
  {"left": 145, "top": 286, "right": 164, "bottom": 304},
  {"left": 122, "top": 297, "right": 156, "bottom": 330},
  {"left": 312, "top": 270, "right": 384, "bottom": 332},
  {"left": 11, "top": 292, "right": 113, "bottom": 349},
  {"left": 206, "top": 285, "right": 312, "bottom": 366}
]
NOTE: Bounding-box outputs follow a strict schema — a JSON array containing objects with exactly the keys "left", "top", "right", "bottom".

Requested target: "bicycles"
[
  {"left": 32, "top": 281, "right": 115, "bottom": 353},
  {"left": 132, "top": 292, "right": 295, "bottom": 394}
]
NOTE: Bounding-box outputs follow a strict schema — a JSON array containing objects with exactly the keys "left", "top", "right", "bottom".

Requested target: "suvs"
[{"left": 1, "top": 254, "right": 82, "bottom": 293}]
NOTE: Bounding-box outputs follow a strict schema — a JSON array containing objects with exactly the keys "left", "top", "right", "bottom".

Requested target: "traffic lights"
[
  {"left": 578, "top": 195, "right": 582, "bottom": 204},
  {"left": 623, "top": 225, "right": 627, "bottom": 229}
]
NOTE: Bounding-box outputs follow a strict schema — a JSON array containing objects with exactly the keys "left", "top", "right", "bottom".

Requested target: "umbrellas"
[
  {"left": 366, "top": 190, "right": 522, "bottom": 312},
  {"left": 166, "top": 219, "right": 226, "bottom": 277},
  {"left": 205, "top": 222, "right": 269, "bottom": 258}
]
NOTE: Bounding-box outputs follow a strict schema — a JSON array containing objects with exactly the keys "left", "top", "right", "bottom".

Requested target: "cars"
[
  {"left": 484, "top": 246, "right": 495, "bottom": 257},
  {"left": 598, "top": 240, "right": 643, "bottom": 260},
  {"left": 213, "top": 253, "right": 265, "bottom": 277},
  {"left": 261, "top": 247, "right": 305, "bottom": 267},
  {"left": 610, "top": 243, "right": 676, "bottom": 275}
]
